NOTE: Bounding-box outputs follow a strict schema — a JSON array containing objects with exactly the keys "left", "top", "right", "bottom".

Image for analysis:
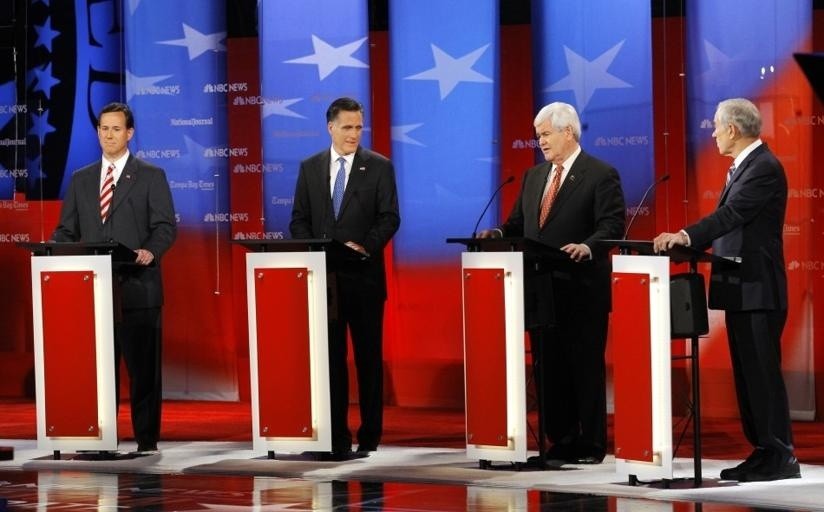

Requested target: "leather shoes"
[
  {"left": 719, "top": 445, "right": 803, "bottom": 484},
  {"left": 136, "top": 439, "right": 160, "bottom": 455},
  {"left": 306, "top": 436, "right": 387, "bottom": 460},
  {"left": 525, "top": 436, "right": 611, "bottom": 467}
]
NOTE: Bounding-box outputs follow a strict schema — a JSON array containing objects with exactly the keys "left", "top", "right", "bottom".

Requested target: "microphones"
[
  {"left": 471, "top": 175, "right": 516, "bottom": 240},
  {"left": 622, "top": 172, "right": 670, "bottom": 240},
  {"left": 322, "top": 175, "right": 331, "bottom": 238},
  {"left": 109, "top": 184, "right": 116, "bottom": 242}
]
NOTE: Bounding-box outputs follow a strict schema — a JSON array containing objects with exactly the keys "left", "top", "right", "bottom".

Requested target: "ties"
[
  {"left": 331, "top": 156, "right": 347, "bottom": 223},
  {"left": 98, "top": 163, "right": 116, "bottom": 226},
  {"left": 538, "top": 166, "right": 565, "bottom": 230},
  {"left": 725, "top": 162, "right": 737, "bottom": 189}
]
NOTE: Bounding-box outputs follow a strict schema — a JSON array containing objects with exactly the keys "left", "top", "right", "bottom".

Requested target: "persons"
[
  {"left": 473, "top": 100, "right": 629, "bottom": 467},
  {"left": 282, "top": 93, "right": 403, "bottom": 460},
  {"left": 650, "top": 94, "right": 807, "bottom": 486},
  {"left": 36, "top": 101, "right": 178, "bottom": 457}
]
